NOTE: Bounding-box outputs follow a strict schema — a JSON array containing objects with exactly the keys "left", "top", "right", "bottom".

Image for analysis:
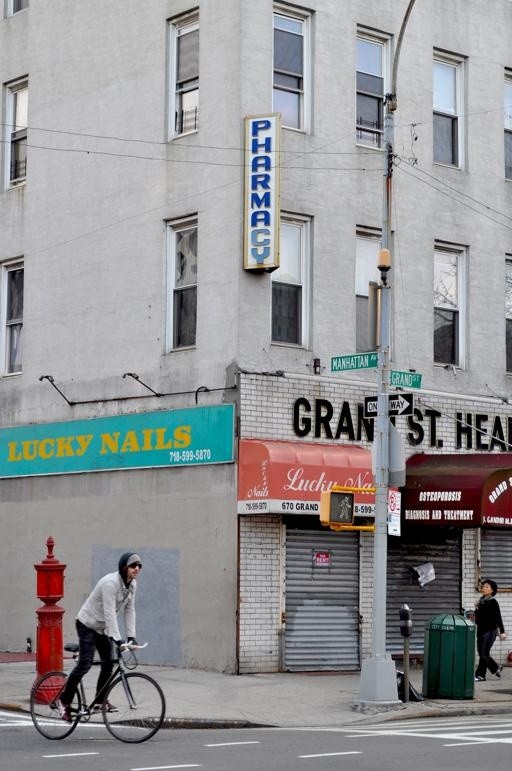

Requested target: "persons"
[
  {"left": 51, "top": 553, "right": 143, "bottom": 722},
  {"left": 467, "top": 580, "right": 506, "bottom": 681}
]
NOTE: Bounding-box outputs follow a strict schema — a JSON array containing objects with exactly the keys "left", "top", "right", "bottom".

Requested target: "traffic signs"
[
  {"left": 389, "top": 370, "right": 421, "bottom": 388},
  {"left": 363, "top": 393, "right": 414, "bottom": 418},
  {"left": 330, "top": 352, "right": 377, "bottom": 371}
]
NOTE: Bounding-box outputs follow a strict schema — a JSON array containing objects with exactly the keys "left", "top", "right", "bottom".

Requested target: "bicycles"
[{"left": 29, "top": 639, "right": 165, "bottom": 743}]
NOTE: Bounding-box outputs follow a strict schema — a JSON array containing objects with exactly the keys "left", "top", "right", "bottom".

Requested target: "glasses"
[{"left": 130, "top": 562, "right": 142, "bottom": 568}]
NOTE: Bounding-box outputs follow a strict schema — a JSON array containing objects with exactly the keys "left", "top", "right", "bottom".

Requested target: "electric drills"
[{"left": 422, "top": 612, "right": 478, "bottom": 698}]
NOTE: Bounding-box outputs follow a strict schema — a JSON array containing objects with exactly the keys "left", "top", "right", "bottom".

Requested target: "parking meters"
[{"left": 399, "top": 604, "right": 413, "bottom": 701}]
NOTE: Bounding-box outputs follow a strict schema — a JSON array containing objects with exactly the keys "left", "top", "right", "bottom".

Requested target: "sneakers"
[
  {"left": 474, "top": 676, "right": 486, "bottom": 681},
  {"left": 92, "top": 703, "right": 119, "bottom": 712},
  {"left": 495, "top": 665, "right": 503, "bottom": 680},
  {"left": 50, "top": 699, "right": 73, "bottom": 724}
]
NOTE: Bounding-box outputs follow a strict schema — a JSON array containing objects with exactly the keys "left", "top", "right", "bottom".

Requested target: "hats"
[{"left": 127, "top": 554, "right": 141, "bottom": 567}]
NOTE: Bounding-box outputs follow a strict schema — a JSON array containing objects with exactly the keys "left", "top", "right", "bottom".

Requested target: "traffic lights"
[{"left": 319, "top": 491, "right": 354, "bottom": 525}]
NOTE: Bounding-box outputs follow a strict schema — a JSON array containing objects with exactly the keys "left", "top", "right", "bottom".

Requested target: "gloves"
[{"left": 117, "top": 637, "right": 138, "bottom": 652}]
